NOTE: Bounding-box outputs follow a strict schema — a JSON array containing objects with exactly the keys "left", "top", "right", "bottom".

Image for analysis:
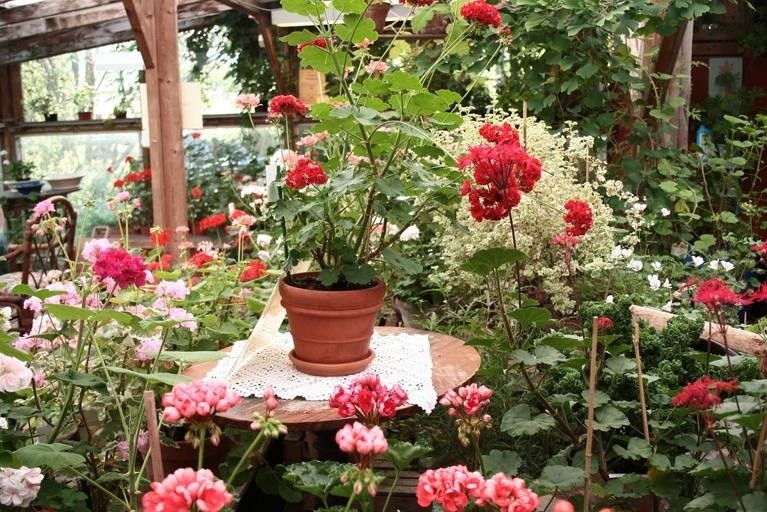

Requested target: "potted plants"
[
  {"left": 1, "top": 159, "right": 44, "bottom": 193},
  {"left": 111, "top": 96, "right": 134, "bottom": 119},
  {"left": 27, "top": 96, "right": 57, "bottom": 122},
  {"left": 74, "top": 86, "right": 97, "bottom": 120}
]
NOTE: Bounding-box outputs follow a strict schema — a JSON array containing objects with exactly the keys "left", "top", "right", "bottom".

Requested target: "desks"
[
  {"left": 1, "top": 185, "right": 82, "bottom": 273},
  {"left": 177, "top": 323, "right": 481, "bottom": 509}
]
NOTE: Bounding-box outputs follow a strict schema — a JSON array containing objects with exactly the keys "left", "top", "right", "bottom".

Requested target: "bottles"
[{"left": 694, "top": 123, "right": 712, "bottom": 153}]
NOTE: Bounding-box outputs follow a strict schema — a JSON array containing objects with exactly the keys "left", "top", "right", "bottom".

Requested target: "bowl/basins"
[
  {"left": 14, "top": 184, "right": 45, "bottom": 195},
  {"left": 48, "top": 177, "right": 85, "bottom": 189}
]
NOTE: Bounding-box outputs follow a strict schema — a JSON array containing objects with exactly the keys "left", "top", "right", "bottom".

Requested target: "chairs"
[{"left": 0, "top": 196, "right": 77, "bottom": 339}]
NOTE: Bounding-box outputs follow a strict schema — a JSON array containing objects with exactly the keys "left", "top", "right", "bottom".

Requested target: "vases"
[{"left": 277, "top": 267, "right": 387, "bottom": 376}]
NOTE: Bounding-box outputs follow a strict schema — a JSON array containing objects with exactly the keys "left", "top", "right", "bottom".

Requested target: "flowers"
[{"left": 258, "top": 1, "right": 546, "bottom": 282}]
